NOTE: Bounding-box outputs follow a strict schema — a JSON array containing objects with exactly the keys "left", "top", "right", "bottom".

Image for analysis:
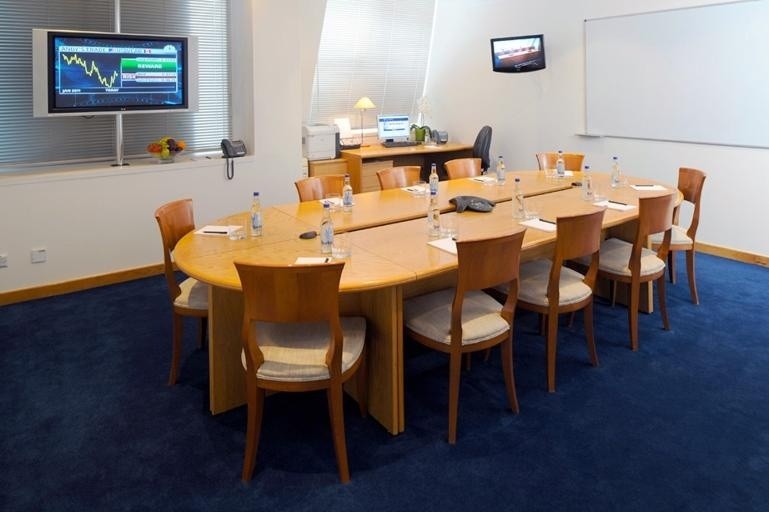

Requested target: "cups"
[
  {"left": 228, "top": 216, "right": 246, "bottom": 241},
  {"left": 525, "top": 198, "right": 541, "bottom": 217},
  {"left": 413, "top": 180, "right": 427, "bottom": 196},
  {"left": 440, "top": 215, "right": 459, "bottom": 237},
  {"left": 328, "top": 192, "right": 341, "bottom": 210},
  {"left": 332, "top": 231, "right": 354, "bottom": 258}
]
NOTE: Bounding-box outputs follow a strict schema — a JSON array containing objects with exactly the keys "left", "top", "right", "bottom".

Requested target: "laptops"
[{"left": 376, "top": 114, "right": 422, "bottom": 148}]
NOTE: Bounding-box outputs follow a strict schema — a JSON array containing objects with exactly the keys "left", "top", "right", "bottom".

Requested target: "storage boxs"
[{"left": 302, "top": 126, "right": 338, "bottom": 161}]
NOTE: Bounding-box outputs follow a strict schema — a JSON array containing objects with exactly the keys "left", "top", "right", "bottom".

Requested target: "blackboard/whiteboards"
[{"left": 583, "top": 0, "right": 769, "bottom": 149}]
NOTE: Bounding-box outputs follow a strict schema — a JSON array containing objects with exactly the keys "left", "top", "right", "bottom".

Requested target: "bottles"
[
  {"left": 429, "top": 162, "right": 440, "bottom": 193},
  {"left": 556, "top": 150, "right": 565, "bottom": 178},
  {"left": 498, "top": 155, "right": 506, "bottom": 185},
  {"left": 510, "top": 178, "right": 524, "bottom": 219},
  {"left": 610, "top": 155, "right": 621, "bottom": 193},
  {"left": 583, "top": 165, "right": 594, "bottom": 201},
  {"left": 248, "top": 192, "right": 263, "bottom": 237},
  {"left": 342, "top": 174, "right": 354, "bottom": 211},
  {"left": 320, "top": 202, "right": 333, "bottom": 252},
  {"left": 428, "top": 191, "right": 439, "bottom": 236}
]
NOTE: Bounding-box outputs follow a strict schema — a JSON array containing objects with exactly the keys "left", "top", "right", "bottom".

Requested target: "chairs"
[
  {"left": 233, "top": 260, "right": 367, "bottom": 484},
  {"left": 174, "top": 141, "right": 685, "bottom": 445},
  {"left": 652, "top": 167, "right": 707, "bottom": 304},
  {"left": 473, "top": 125, "right": 493, "bottom": 174},
  {"left": 155, "top": 199, "right": 208, "bottom": 387}
]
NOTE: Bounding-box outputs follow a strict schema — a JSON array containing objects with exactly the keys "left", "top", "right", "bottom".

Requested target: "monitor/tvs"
[
  {"left": 489, "top": 33, "right": 547, "bottom": 74},
  {"left": 30, "top": 27, "right": 200, "bottom": 119}
]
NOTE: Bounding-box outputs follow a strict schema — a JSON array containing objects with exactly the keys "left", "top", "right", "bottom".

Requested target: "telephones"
[
  {"left": 221, "top": 139, "right": 247, "bottom": 158},
  {"left": 449, "top": 196, "right": 495, "bottom": 213}
]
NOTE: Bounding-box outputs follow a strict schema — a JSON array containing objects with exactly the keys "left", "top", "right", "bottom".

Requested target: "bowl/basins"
[{"left": 147, "top": 145, "right": 184, "bottom": 161}]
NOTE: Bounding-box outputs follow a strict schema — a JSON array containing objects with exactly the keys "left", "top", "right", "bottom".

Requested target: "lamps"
[{"left": 354, "top": 95, "right": 377, "bottom": 148}]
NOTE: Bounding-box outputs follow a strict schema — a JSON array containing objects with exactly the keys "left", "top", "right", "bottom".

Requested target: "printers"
[{"left": 302, "top": 120, "right": 341, "bottom": 161}]
